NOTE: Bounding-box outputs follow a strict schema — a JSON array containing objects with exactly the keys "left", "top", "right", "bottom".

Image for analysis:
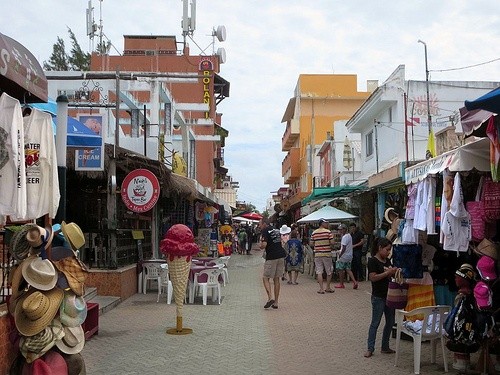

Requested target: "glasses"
[{"left": 352, "top": 227, "right": 355, "bottom": 228}]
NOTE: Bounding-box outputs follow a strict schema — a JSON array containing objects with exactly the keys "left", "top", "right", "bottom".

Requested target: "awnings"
[
  {"left": 170, "top": 173, "right": 236, "bottom": 219},
  {"left": 404, "top": 136, "right": 491, "bottom": 186},
  {"left": 300, "top": 183, "right": 371, "bottom": 206}
]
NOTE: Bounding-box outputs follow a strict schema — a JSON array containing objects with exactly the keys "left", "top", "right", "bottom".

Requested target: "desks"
[
  {"left": 189, "top": 266, "right": 225, "bottom": 303},
  {"left": 193, "top": 256, "right": 216, "bottom": 265}
]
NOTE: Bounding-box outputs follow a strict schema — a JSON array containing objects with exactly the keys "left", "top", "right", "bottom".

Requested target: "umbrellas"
[
  {"left": 232, "top": 211, "right": 264, "bottom": 223},
  {"left": 297, "top": 204, "right": 359, "bottom": 224},
  {"left": 23, "top": 100, "right": 102, "bottom": 223}
]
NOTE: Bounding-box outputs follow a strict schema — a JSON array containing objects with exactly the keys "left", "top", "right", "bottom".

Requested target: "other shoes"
[
  {"left": 317, "top": 290, "right": 324, "bottom": 294},
  {"left": 287, "top": 281, "right": 292, "bottom": 284},
  {"left": 325, "top": 288, "right": 335, "bottom": 293},
  {"left": 272, "top": 305, "right": 278, "bottom": 309},
  {"left": 381, "top": 348, "right": 396, "bottom": 353},
  {"left": 264, "top": 298, "right": 275, "bottom": 308},
  {"left": 353, "top": 282, "right": 358, "bottom": 289},
  {"left": 293, "top": 281, "right": 298, "bottom": 285},
  {"left": 285, "top": 276, "right": 287, "bottom": 280},
  {"left": 282, "top": 273, "right": 285, "bottom": 280},
  {"left": 364, "top": 351, "right": 374, "bottom": 357},
  {"left": 335, "top": 284, "right": 345, "bottom": 288}
]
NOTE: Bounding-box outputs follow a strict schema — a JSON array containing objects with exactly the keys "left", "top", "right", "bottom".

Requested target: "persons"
[
  {"left": 348, "top": 223, "right": 365, "bottom": 282},
  {"left": 363, "top": 236, "right": 405, "bottom": 358},
  {"left": 443, "top": 263, "right": 487, "bottom": 375},
  {"left": 334, "top": 225, "right": 358, "bottom": 289},
  {"left": 186, "top": 219, "right": 355, "bottom": 256},
  {"left": 158, "top": 214, "right": 172, "bottom": 259},
  {"left": 283, "top": 230, "right": 305, "bottom": 284},
  {"left": 279, "top": 224, "right": 292, "bottom": 280},
  {"left": 259, "top": 217, "right": 287, "bottom": 309},
  {"left": 309, "top": 220, "right": 335, "bottom": 293}
]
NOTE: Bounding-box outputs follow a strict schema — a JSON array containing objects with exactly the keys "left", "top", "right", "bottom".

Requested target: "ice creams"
[{"left": 160, "top": 223, "right": 200, "bottom": 317}]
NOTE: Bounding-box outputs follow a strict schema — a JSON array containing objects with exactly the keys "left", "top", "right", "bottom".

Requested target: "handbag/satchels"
[
  {"left": 260, "top": 250, "right": 267, "bottom": 263},
  {"left": 386, "top": 268, "right": 408, "bottom": 309},
  {"left": 246, "top": 242, "right": 248, "bottom": 250}
]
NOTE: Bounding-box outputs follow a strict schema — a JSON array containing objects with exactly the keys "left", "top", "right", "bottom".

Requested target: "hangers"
[{"left": 21, "top": 90, "right": 32, "bottom": 116}]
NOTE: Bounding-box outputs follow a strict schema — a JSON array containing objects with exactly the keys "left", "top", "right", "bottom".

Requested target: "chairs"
[
  {"left": 394, "top": 306, "right": 450, "bottom": 374},
  {"left": 141, "top": 255, "right": 230, "bottom": 307}
]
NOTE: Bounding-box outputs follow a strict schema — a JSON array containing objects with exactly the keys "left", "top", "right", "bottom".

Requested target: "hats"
[
  {"left": 456, "top": 264, "right": 474, "bottom": 282},
  {"left": 474, "top": 238, "right": 499, "bottom": 261},
  {"left": 473, "top": 283, "right": 493, "bottom": 311},
  {"left": 318, "top": 218, "right": 328, "bottom": 224},
  {"left": 476, "top": 256, "right": 498, "bottom": 281},
  {"left": 338, "top": 224, "right": 347, "bottom": 229},
  {"left": 279, "top": 225, "right": 291, "bottom": 234},
  {"left": 4, "top": 221, "right": 89, "bottom": 375}
]
